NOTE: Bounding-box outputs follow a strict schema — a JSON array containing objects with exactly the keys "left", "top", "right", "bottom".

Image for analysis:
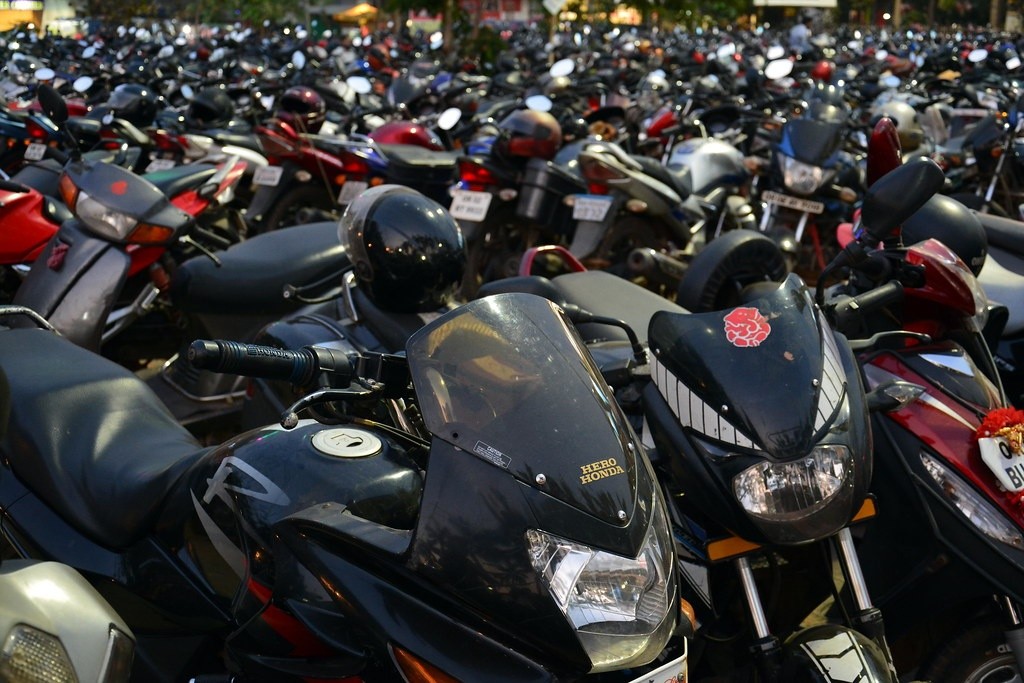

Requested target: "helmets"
[
  {"left": 639, "top": 76, "right": 668, "bottom": 97},
  {"left": 184, "top": 88, "right": 233, "bottom": 131},
  {"left": 347, "top": 189, "right": 465, "bottom": 315},
  {"left": 107, "top": 83, "right": 156, "bottom": 127},
  {"left": 546, "top": 77, "right": 569, "bottom": 96},
  {"left": 274, "top": 87, "right": 326, "bottom": 133},
  {"left": 901, "top": 193, "right": 986, "bottom": 278},
  {"left": 813, "top": 61, "right": 834, "bottom": 83},
  {"left": 872, "top": 101, "right": 916, "bottom": 134},
  {"left": 499, "top": 109, "right": 563, "bottom": 160}
]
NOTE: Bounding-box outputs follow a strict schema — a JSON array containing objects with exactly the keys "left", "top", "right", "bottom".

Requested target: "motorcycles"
[{"left": 0, "top": 0, "right": 1024, "bottom": 683}]
